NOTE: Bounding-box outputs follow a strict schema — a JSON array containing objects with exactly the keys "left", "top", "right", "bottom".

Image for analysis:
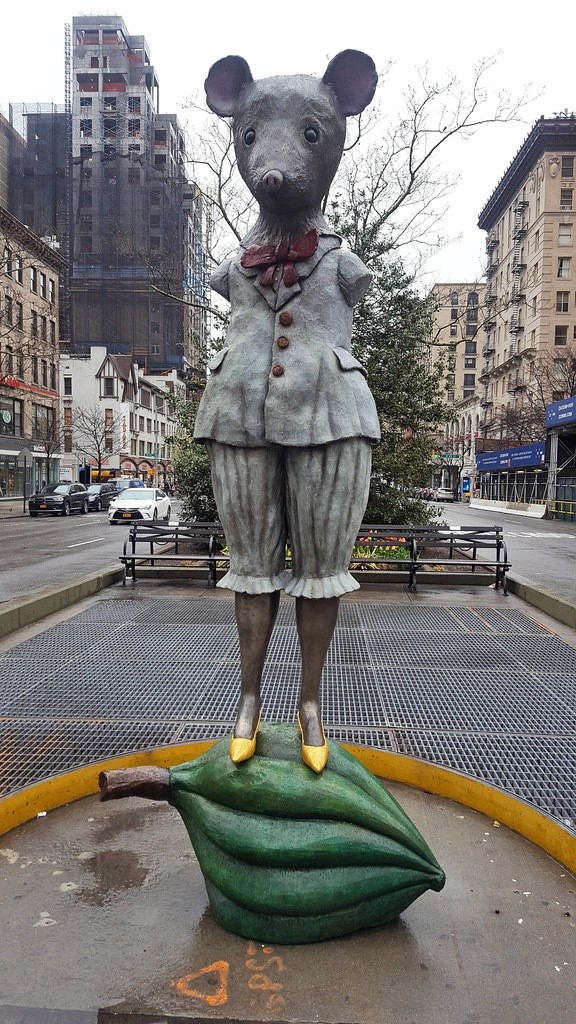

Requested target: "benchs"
[{"left": 120, "top": 517, "right": 511, "bottom": 593}]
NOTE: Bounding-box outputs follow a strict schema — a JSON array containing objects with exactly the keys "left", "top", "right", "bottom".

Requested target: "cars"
[
  {"left": 107, "top": 479, "right": 145, "bottom": 493},
  {"left": 407, "top": 487, "right": 455, "bottom": 503},
  {"left": 28, "top": 483, "right": 89, "bottom": 517},
  {"left": 82, "top": 483, "right": 117, "bottom": 512},
  {"left": 108, "top": 488, "right": 172, "bottom": 525}
]
